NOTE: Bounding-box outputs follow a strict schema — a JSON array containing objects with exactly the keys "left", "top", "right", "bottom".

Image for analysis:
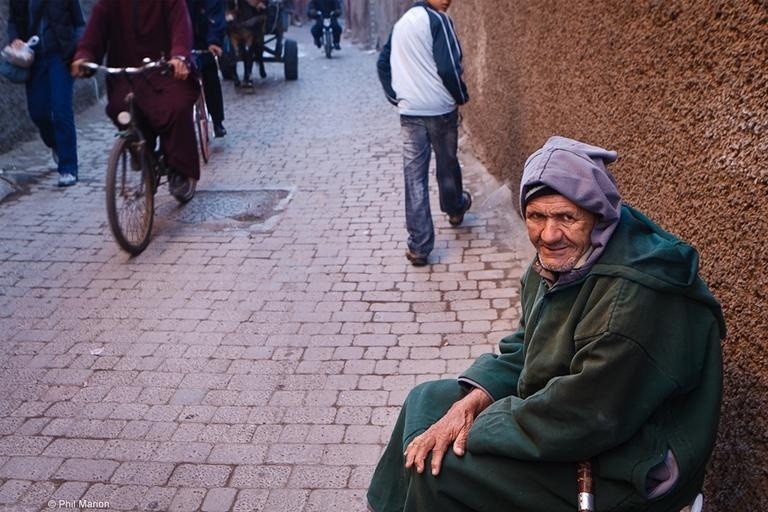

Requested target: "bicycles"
[{"left": 79, "top": 49, "right": 213, "bottom": 256}]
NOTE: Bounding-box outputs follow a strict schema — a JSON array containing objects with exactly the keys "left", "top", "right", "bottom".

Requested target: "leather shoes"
[{"left": 214, "top": 121, "right": 226, "bottom": 134}]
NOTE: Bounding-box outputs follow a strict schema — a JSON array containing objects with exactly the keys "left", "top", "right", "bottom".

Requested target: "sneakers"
[
  {"left": 335, "top": 42, "right": 339, "bottom": 49},
  {"left": 175, "top": 177, "right": 198, "bottom": 202},
  {"left": 316, "top": 40, "right": 321, "bottom": 47},
  {"left": 448, "top": 190, "right": 472, "bottom": 226},
  {"left": 57, "top": 172, "right": 78, "bottom": 187},
  {"left": 261, "top": 66, "right": 267, "bottom": 77},
  {"left": 406, "top": 248, "right": 428, "bottom": 265}
]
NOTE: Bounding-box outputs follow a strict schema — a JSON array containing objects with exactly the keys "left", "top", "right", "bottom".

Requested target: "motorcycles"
[{"left": 314, "top": 8, "right": 337, "bottom": 59}]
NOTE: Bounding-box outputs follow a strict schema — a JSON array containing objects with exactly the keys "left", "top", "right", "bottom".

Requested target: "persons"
[
  {"left": 376, "top": 0, "right": 472, "bottom": 266},
  {"left": 307, "top": 0, "right": 342, "bottom": 50},
  {"left": 70, "top": 0, "right": 201, "bottom": 195},
  {"left": 7, "top": 0, "right": 88, "bottom": 186},
  {"left": 366, "top": 135, "right": 728, "bottom": 512},
  {"left": 184, "top": 0, "right": 228, "bottom": 138}
]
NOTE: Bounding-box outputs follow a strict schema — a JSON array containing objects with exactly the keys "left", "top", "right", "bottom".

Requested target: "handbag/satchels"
[{"left": 0, "top": 61, "right": 31, "bottom": 82}]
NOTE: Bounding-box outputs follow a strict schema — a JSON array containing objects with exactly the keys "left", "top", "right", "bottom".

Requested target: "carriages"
[{"left": 209, "top": 0, "right": 298, "bottom": 95}]
